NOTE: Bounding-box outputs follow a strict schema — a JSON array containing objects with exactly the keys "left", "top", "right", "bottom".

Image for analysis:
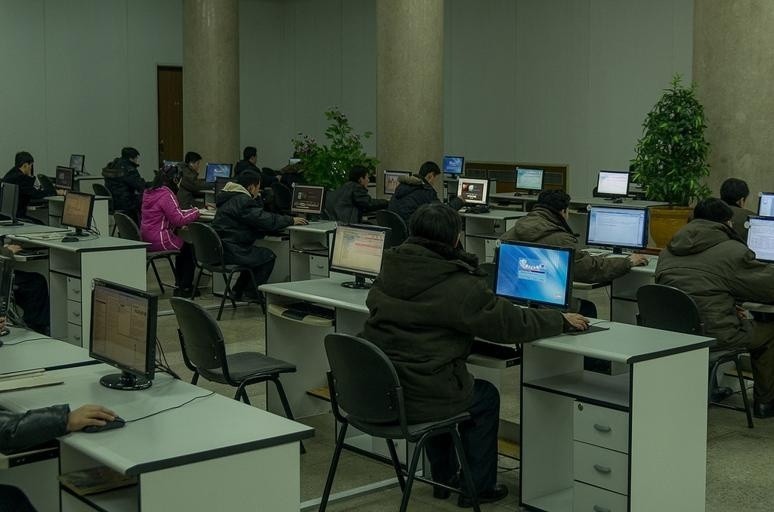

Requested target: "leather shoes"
[
  {"left": 434, "top": 472, "right": 461, "bottom": 500},
  {"left": 458, "top": 484, "right": 509, "bottom": 508},
  {"left": 173, "top": 288, "right": 182, "bottom": 297},
  {"left": 241, "top": 289, "right": 266, "bottom": 302},
  {"left": 711, "top": 386, "right": 733, "bottom": 403},
  {"left": 753, "top": 401, "right": 774, "bottom": 419},
  {"left": 181, "top": 287, "right": 201, "bottom": 298},
  {"left": 229, "top": 289, "right": 247, "bottom": 301}
]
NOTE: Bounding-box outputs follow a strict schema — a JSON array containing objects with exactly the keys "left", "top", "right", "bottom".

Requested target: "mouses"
[
  {"left": 62, "top": 237, "right": 79, "bottom": 242},
  {"left": 636, "top": 260, "right": 649, "bottom": 266},
  {"left": 297, "top": 221, "right": 309, "bottom": 225},
  {"left": 83, "top": 416, "right": 125, "bottom": 433},
  {"left": 568, "top": 323, "right": 589, "bottom": 333},
  {"left": 514, "top": 192, "right": 521, "bottom": 197}
]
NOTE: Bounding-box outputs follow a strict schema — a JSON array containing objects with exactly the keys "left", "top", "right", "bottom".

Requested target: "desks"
[
  {"left": 26, "top": 194, "right": 112, "bottom": 236},
  {"left": 565, "top": 197, "right": 670, "bottom": 236},
  {"left": 0, "top": 220, "right": 153, "bottom": 350},
  {"left": 259, "top": 275, "right": 718, "bottom": 512},
  {"left": 572, "top": 248, "right": 774, "bottom": 376},
  {"left": 362, "top": 177, "right": 540, "bottom": 264},
  {"left": 0, "top": 324, "right": 315, "bottom": 512},
  {"left": 181, "top": 208, "right": 337, "bottom": 302}
]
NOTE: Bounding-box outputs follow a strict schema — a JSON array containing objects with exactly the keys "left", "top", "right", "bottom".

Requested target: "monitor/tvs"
[
  {"left": 743, "top": 215, "right": 774, "bottom": 265},
  {"left": 89, "top": 277, "right": 158, "bottom": 391},
  {"left": 0, "top": 182, "right": 25, "bottom": 226},
  {"left": 597, "top": 169, "right": 630, "bottom": 200},
  {"left": 443, "top": 156, "right": 464, "bottom": 178},
  {"left": 55, "top": 153, "right": 86, "bottom": 191},
  {"left": 494, "top": 239, "right": 576, "bottom": 310},
  {"left": 515, "top": 167, "right": 544, "bottom": 195},
  {"left": 291, "top": 184, "right": 326, "bottom": 219},
  {"left": 328, "top": 222, "right": 392, "bottom": 290},
  {"left": 756, "top": 191, "right": 774, "bottom": 216},
  {"left": 61, "top": 190, "right": 95, "bottom": 237},
  {"left": 457, "top": 177, "right": 491, "bottom": 206},
  {"left": 585, "top": 204, "right": 650, "bottom": 257},
  {"left": 383, "top": 170, "right": 412, "bottom": 195},
  {"left": 205, "top": 163, "right": 233, "bottom": 183}
]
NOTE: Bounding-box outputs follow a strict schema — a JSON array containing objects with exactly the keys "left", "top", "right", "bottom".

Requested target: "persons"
[
  {"left": 719, "top": 179, "right": 762, "bottom": 245},
  {"left": 2, "top": 150, "right": 65, "bottom": 226},
  {"left": 333, "top": 165, "right": 390, "bottom": 226},
  {"left": 386, "top": 160, "right": 466, "bottom": 235},
  {"left": 141, "top": 165, "right": 200, "bottom": 299},
  {"left": 177, "top": 145, "right": 306, "bottom": 210},
  {"left": 495, "top": 187, "right": 649, "bottom": 320},
  {"left": 655, "top": 196, "right": 774, "bottom": 418},
  {"left": 0, "top": 397, "right": 117, "bottom": 512},
  {"left": 207, "top": 169, "right": 309, "bottom": 303},
  {"left": 101, "top": 147, "right": 152, "bottom": 237},
  {"left": 352, "top": 203, "right": 591, "bottom": 509}
]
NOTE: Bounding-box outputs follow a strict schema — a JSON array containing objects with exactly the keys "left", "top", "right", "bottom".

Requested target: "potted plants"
[{"left": 631, "top": 73, "right": 714, "bottom": 247}]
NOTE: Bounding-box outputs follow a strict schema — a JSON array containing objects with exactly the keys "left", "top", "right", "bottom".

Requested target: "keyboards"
[
  {"left": 16, "top": 249, "right": 37, "bottom": 256},
  {"left": 282, "top": 301, "right": 336, "bottom": 320}
]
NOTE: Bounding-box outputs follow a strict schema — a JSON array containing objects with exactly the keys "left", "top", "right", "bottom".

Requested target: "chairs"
[
  {"left": 635, "top": 283, "right": 754, "bottom": 442},
  {"left": 168, "top": 296, "right": 306, "bottom": 455},
  {"left": 114, "top": 211, "right": 185, "bottom": 297},
  {"left": 37, "top": 174, "right": 215, "bottom": 236},
  {"left": 318, "top": 333, "right": 482, "bottom": 511},
  {"left": 188, "top": 222, "right": 266, "bottom": 320},
  {"left": 478, "top": 264, "right": 527, "bottom": 306}
]
